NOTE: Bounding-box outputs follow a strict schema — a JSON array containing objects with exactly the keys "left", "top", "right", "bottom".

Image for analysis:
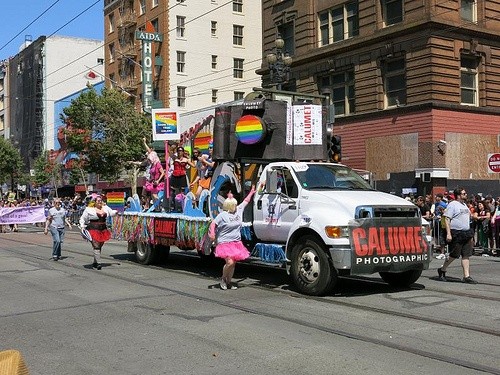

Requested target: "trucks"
[{"left": 116, "top": 162, "right": 432, "bottom": 297}]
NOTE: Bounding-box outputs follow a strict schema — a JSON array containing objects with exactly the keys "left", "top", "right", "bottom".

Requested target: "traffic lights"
[{"left": 331, "top": 135, "right": 341, "bottom": 162}]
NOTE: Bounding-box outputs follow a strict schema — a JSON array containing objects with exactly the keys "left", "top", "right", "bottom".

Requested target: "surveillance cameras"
[{"left": 439, "top": 140, "right": 446, "bottom": 144}]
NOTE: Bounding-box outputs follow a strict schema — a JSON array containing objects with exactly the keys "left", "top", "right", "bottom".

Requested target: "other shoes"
[
  {"left": 437, "top": 268, "right": 448, "bottom": 282},
  {"left": 53, "top": 256, "right": 63, "bottom": 262},
  {"left": 226, "top": 283, "right": 237, "bottom": 290},
  {"left": 219, "top": 278, "right": 228, "bottom": 290},
  {"left": 92, "top": 262, "right": 102, "bottom": 270},
  {"left": 435, "top": 253, "right": 449, "bottom": 260},
  {"left": 462, "top": 276, "right": 478, "bottom": 284}
]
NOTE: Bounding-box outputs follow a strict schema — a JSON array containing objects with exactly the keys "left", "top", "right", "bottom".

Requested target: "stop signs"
[{"left": 488, "top": 154, "right": 500, "bottom": 172}]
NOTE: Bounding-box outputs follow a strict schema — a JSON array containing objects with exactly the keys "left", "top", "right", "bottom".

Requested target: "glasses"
[{"left": 461, "top": 192, "right": 467, "bottom": 196}]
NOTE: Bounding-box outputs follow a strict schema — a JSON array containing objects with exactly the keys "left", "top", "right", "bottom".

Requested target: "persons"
[
  {"left": 0, "top": 137, "right": 215, "bottom": 269},
  {"left": 400, "top": 188, "right": 500, "bottom": 283},
  {"left": 208, "top": 185, "right": 257, "bottom": 290}
]
